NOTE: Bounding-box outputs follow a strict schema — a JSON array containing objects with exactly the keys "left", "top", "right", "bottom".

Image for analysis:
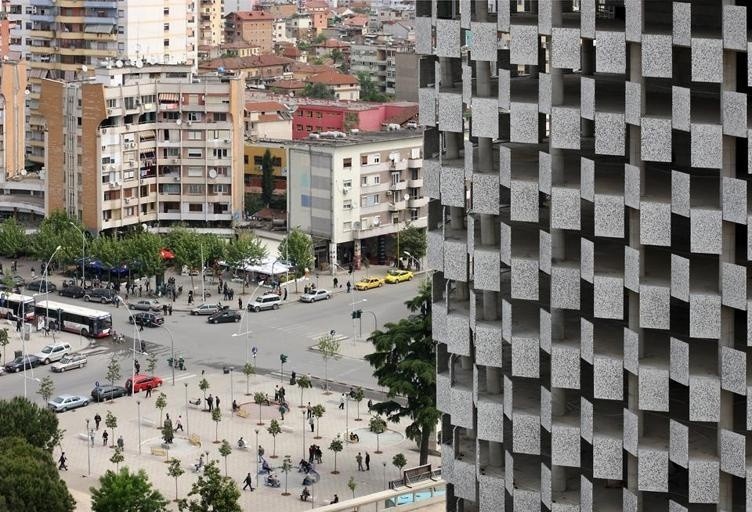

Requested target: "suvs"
[
  {"left": 38, "top": 343, "right": 72, "bottom": 365},
  {"left": 130, "top": 312, "right": 165, "bottom": 327},
  {"left": 248, "top": 294, "right": 282, "bottom": 312}
]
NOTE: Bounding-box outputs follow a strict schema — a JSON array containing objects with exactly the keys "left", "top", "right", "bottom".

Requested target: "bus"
[
  {"left": 1, "top": 291, "right": 34, "bottom": 323},
  {"left": 36, "top": 300, "right": 115, "bottom": 338}
]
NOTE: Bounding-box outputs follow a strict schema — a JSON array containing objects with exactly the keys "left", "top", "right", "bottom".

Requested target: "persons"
[
  {"left": 127, "top": 340, "right": 153, "bottom": 397},
  {"left": 176, "top": 393, "right": 273, "bottom": 492},
  {"left": 274, "top": 371, "right": 371, "bottom": 504},
  {"left": 163, "top": 415, "right": 174, "bottom": 443},
  {"left": 12, "top": 258, "right": 150, "bottom": 308},
  {"left": 16, "top": 319, "right": 50, "bottom": 334},
  {"left": 333, "top": 258, "right": 371, "bottom": 293},
  {"left": 179, "top": 357, "right": 185, "bottom": 370},
  {"left": 159, "top": 275, "right": 245, "bottom": 316},
  {"left": 272, "top": 279, "right": 316, "bottom": 300},
  {"left": 57, "top": 414, "right": 125, "bottom": 471},
  {"left": 113, "top": 318, "right": 143, "bottom": 342}
]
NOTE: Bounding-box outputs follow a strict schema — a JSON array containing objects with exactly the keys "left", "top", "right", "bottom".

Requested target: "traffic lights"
[
  {"left": 351, "top": 312, "right": 354, "bottom": 318},
  {"left": 359, "top": 310, "right": 363, "bottom": 318}
]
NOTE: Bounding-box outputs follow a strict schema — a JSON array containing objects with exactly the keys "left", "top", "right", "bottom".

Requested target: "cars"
[
  {"left": 0, "top": 270, "right": 115, "bottom": 303},
  {"left": 126, "top": 374, "right": 163, "bottom": 392},
  {"left": 301, "top": 289, "right": 332, "bottom": 303},
  {"left": 209, "top": 309, "right": 241, "bottom": 323},
  {"left": 6, "top": 354, "right": 41, "bottom": 372},
  {"left": 47, "top": 393, "right": 91, "bottom": 413},
  {"left": 130, "top": 300, "right": 160, "bottom": 310},
  {"left": 384, "top": 269, "right": 413, "bottom": 284},
  {"left": 205, "top": 268, "right": 214, "bottom": 276},
  {"left": 191, "top": 302, "right": 221, "bottom": 315},
  {"left": 52, "top": 354, "right": 89, "bottom": 372},
  {"left": 1, "top": 365, "right": 6, "bottom": 374},
  {"left": 354, "top": 277, "right": 385, "bottom": 291},
  {"left": 92, "top": 383, "right": 127, "bottom": 402}
]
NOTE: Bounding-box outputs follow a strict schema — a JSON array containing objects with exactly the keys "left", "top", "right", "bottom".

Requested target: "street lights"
[
  {"left": 201, "top": 390, "right": 387, "bottom": 511},
  {"left": 114, "top": 229, "right": 124, "bottom": 284},
  {"left": 67, "top": 222, "right": 92, "bottom": 258},
  {"left": 401, "top": 248, "right": 431, "bottom": 286},
  {"left": 111, "top": 294, "right": 142, "bottom": 354},
  {"left": 183, "top": 381, "right": 190, "bottom": 435},
  {"left": 4, "top": 318, "right": 34, "bottom": 378},
  {"left": 39, "top": 244, "right": 63, "bottom": 302},
  {"left": 86, "top": 416, "right": 93, "bottom": 474},
  {"left": 286, "top": 222, "right": 303, "bottom": 292},
  {"left": 238, "top": 279, "right": 265, "bottom": 395},
  {"left": 229, "top": 365, "right": 235, "bottom": 404},
  {"left": 135, "top": 399, "right": 143, "bottom": 452}
]
